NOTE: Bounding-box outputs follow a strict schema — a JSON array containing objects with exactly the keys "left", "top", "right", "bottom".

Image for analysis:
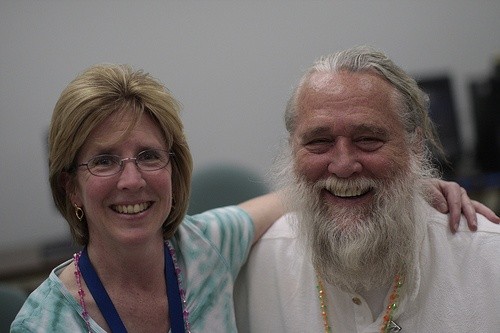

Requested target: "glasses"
[{"left": 74, "top": 148, "right": 175, "bottom": 178}]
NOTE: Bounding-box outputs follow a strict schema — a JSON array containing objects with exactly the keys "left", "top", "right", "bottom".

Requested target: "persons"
[
  {"left": 10, "top": 63, "right": 500, "bottom": 332},
  {"left": 234, "top": 46, "right": 500, "bottom": 331}
]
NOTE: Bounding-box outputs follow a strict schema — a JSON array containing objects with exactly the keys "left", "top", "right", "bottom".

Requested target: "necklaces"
[
  {"left": 317, "top": 266, "right": 402, "bottom": 331},
  {"left": 73, "top": 240, "right": 191, "bottom": 333}
]
